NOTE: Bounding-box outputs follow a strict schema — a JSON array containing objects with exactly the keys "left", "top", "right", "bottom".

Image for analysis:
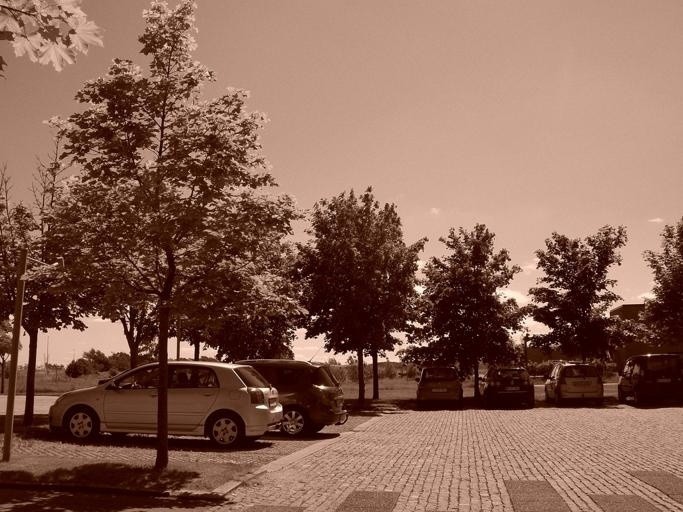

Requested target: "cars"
[
  {"left": 415, "top": 367, "right": 463, "bottom": 406},
  {"left": 618, "top": 353, "right": 683, "bottom": 404},
  {"left": 544, "top": 362, "right": 604, "bottom": 403},
  {"left": 479, "top": 368, "right": 534, "bottom": 408}
]
